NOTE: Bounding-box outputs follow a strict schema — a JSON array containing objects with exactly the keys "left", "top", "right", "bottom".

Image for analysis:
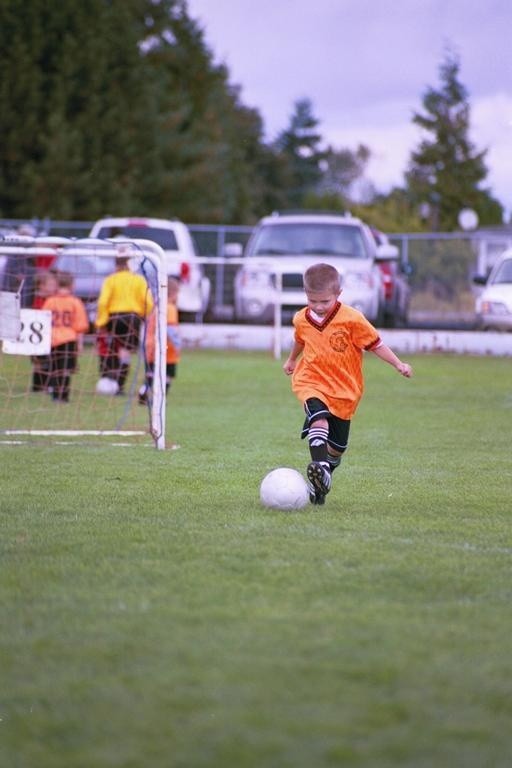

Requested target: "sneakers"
[{"left": 307, "top": 461, "right": 331, "bottom": 505}]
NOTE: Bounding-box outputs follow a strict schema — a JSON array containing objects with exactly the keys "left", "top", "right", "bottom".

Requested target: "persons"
[
  {"left": 280, "top": 262, "right": 413, "bottom": 508},
  {"left": 26, "top": 253, "right": 184, "bottom": 408}
]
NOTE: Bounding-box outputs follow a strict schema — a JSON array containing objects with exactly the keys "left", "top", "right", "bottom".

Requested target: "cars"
[
  {"left": 89, "top": 214, "right": 212, "bottom": 323},
  {"left": 221, "top": 209, "right": 415, "bottom": 327},
  {"left": 471, "top": 249, "right": 512, "bottom": 323}
]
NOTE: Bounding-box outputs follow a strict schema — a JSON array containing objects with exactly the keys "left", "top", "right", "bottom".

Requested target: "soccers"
[
  {"left": 259, "top": 467, "right": 311, "bottom": 513},
  {"left": 94, "top": 375, "right": 120, "bottom": 398}
]
